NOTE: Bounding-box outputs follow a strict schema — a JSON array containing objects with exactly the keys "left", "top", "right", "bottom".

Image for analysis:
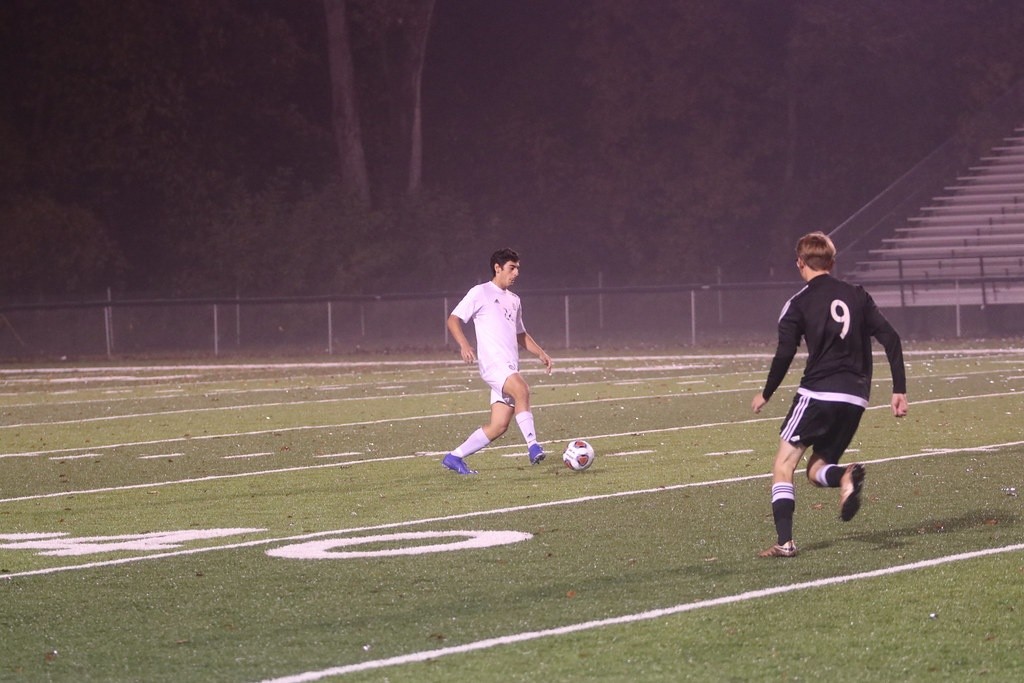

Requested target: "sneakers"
[
  {"left": 528, "top": 444, "right": 545, "bottom": 465},
  {"left": 441, "top": 453, "right": 478, "bottom": 474},
  {"left": 759, "top": 539, "right": 796, "bottom": 557},
  {"left": 839, "top": 464, "right": 865, "bottom": 521}
]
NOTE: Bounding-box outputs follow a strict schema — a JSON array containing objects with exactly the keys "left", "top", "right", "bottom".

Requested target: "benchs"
[{"left": 842, "top": 126, "right": 1024, "bottom": 307}]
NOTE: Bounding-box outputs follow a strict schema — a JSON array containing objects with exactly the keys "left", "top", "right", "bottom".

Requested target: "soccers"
[{"left": 562, "top": 440, "right": 594, "bottom": 472}]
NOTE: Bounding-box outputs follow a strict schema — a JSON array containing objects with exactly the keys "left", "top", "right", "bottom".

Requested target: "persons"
[
  {"left": 444, "top": 248, "right": 552, "bottom": 474},
  {"left": 751, "top": 233, "right": 909, "bottom": 556}
]
